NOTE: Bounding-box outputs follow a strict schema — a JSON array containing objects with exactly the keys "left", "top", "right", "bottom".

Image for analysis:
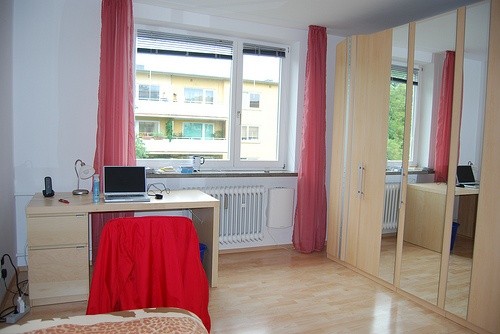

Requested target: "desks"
[
  {"left": 403, "top": 183, "right": 480, "bottom": 257},
  {"left": 26, "top": 191, "right": 221, "bottom": 307}
]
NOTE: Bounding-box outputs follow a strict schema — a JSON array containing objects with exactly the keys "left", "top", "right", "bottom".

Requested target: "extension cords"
[{"left": 6, "top": 305, "right": 31, "bottom": 323}]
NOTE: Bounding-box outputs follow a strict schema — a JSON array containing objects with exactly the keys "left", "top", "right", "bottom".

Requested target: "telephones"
[{"left": 42, "top": 177, "right": 55, "bottom": 197}]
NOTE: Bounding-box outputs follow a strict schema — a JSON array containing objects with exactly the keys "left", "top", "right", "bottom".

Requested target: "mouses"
[
  {"left": 456, "top": 184, "right": 464, "bottom": 188},
  {"left": 155, "top": 194, "right": 163, "bottom": 199}
]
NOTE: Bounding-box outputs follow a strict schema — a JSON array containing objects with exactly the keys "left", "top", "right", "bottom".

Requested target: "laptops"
[
  {"left": 103, "top": 167, "right": 150, "bottom": 202},
  {"left": 456, "top": 165, "right": 481, "bottom": 189}
]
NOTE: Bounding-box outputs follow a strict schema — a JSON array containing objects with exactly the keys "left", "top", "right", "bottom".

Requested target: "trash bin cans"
[
  {"left": 199, "top": 243, "right": 208, "bottom": 263},
  {"left": 450, "top": 222, "right": 460, "bottom": 250}
]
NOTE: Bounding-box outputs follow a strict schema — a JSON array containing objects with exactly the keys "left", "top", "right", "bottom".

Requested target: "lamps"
[{"left": 74, "top": 158, "right": 97, "bottom": 196}]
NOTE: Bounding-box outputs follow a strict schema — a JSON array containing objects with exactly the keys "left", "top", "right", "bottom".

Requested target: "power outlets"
[{"left": 1, "top": 258, "right": 6, "bottom": 277}]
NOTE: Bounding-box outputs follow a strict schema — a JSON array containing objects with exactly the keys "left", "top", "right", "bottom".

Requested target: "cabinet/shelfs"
[{"left": 326, "top": 25, "right": 393, "bottom": 275}]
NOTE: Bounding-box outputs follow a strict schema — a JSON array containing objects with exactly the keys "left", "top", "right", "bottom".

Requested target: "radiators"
[
  {"left": 170, "top": 185, "right": 263, "bottom": 245},
  {"left": 384, "top": 183, "right": 403, "bottom": 231}
]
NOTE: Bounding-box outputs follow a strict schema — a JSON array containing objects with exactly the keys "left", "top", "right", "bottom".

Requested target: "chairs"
[{"left": 95, "top": 216, "right": 211, "bottom": 333}]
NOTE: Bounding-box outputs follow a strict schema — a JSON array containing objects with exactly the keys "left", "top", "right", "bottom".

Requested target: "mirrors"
[{"left": 377, "top": 0, "right": 491, "bottom": 321}]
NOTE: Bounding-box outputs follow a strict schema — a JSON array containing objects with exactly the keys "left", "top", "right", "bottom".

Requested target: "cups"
[{"left": 193, "top": 155, "right": 205, "bottom": 173}]
